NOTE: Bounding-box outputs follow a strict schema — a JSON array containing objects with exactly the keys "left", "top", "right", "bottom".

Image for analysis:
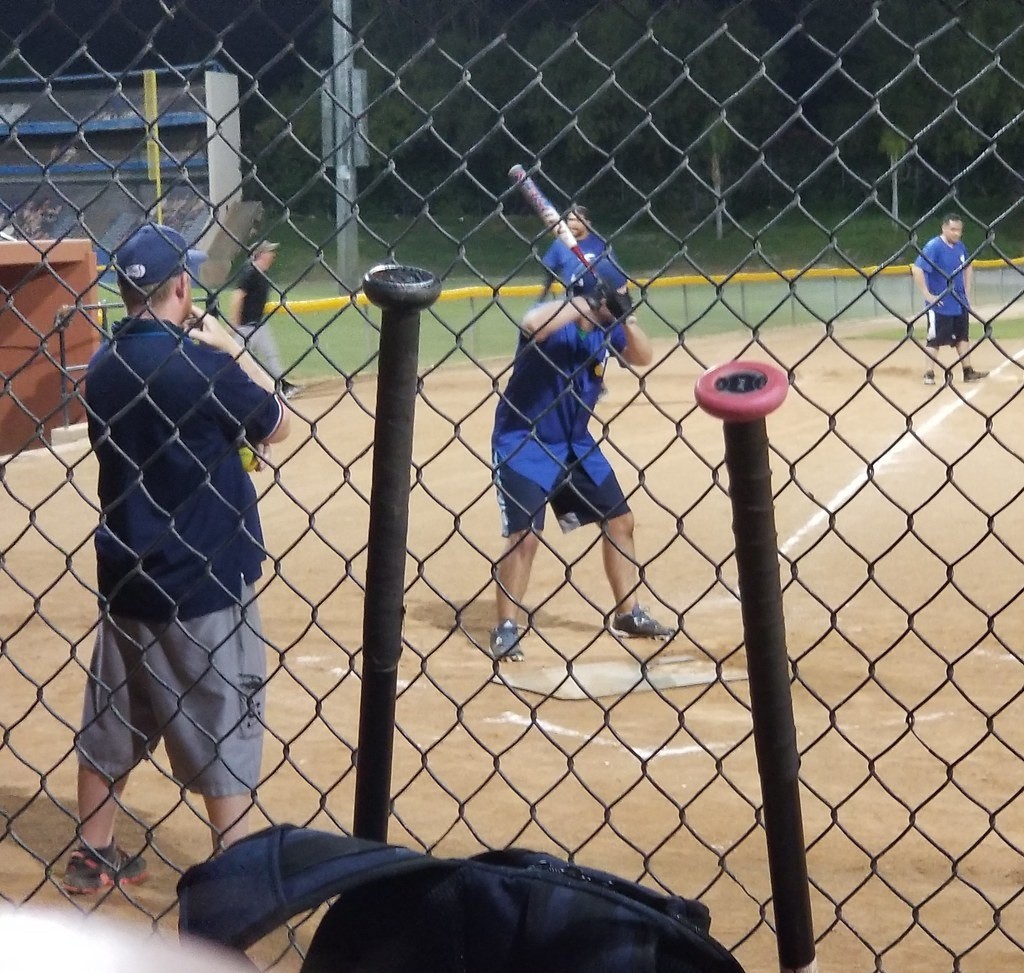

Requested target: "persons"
[
  {"left": 490, "top": 254, "right": 676, "bottom": 662},
  {"left": 536, "top": 205, "right": 619, "bottom": 305},
  {"left": 63, "top": 225, "right": 292, "bottom": 894},
  {"left": 912, "top": 213, "right": 990, "bottom": 384},
  {"left": 228, "top": 239, "right": 305, "bottom": 399}
]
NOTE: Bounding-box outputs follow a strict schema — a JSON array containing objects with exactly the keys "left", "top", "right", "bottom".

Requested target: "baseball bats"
[{"left": 508, "top": 161, "right": 609, "bottom": 292}]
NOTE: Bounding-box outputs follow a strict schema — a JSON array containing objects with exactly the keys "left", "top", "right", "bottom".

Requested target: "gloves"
[
  {"left": 605, "top": 291, "right": 630, "bottom": 322},
  {"left": 581, "top": 279, "right": 611, "bottom": 310}
]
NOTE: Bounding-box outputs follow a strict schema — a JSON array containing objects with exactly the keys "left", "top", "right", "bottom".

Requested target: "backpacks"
[{"left": 176, "top": 824, "right": 748, "bottom": 973}]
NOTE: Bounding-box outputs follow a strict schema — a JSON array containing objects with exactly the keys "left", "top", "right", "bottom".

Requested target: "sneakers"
[
  {"left": 64, "top": 839, "right": 147, "bottom": 894},
  {"left": 489, "top": 626, "right": 524, "bottom": 663},
  {"left": 612, "top": 607, "right": 675, "bottom": 641}
]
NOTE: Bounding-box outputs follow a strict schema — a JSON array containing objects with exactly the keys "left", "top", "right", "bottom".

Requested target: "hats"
[
  {"left": 572, "top": 260, "right": 627, "bottom": 292},
  {"left": 248, "top": 238, "right": 281, "bottom": 256},
  {"left": 116, "top": 225, "right": 207, "bottom": 285}
]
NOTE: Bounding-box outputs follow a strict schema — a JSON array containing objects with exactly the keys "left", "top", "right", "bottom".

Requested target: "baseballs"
[{"left": 239, "top": 447, "right": 258, "bottom": 472}]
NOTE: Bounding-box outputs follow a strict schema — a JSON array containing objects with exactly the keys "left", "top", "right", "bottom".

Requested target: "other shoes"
[
  {"left": 282, "top": 380, "right": 302, "bottom": 399},
  {"left": 964, "top": 369, "right": 989, "bottom": 382},
  {"left": 924, "top": 370, "right": 935, "bottom": 385}
]
